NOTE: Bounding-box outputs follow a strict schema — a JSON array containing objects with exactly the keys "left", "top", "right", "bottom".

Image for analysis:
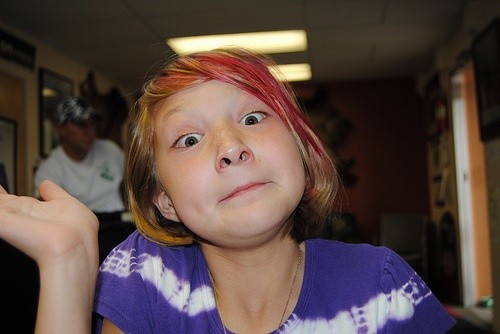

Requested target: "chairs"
[{"left": 375, "top": 213, "right": 427, "bottom": 286}]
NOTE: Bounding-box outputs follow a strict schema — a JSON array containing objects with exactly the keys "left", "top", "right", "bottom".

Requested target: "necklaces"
[{"left": 208, "top": 245, "right": 302, "bottom": 334}]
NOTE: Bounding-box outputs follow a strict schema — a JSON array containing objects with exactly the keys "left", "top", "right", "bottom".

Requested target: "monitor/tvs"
[{"left": 471, "top": 17, "right": 500, "bottom": 143}]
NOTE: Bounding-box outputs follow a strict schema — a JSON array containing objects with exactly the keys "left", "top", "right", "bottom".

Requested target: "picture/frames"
[
  {"left": 36, "top": 64, "right": 77, "bottom": 159},
  {"left": 0, "top": 115, "right": 19, "bottom": 196},
  {"left": 469, "top": 13, "right": 500, "bottom": 141}
]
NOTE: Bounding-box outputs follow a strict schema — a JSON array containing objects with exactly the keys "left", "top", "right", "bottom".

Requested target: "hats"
[{"left": 52, "top": 96, "right": 104, "bottom": 124}]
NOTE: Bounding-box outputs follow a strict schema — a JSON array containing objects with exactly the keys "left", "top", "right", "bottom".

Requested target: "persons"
[
  {"left": 33, "top": 97, "right": 127, "bottom": 266},
  {"left": 0, "top": 49, "right": 458, "bottom": 334}
]
